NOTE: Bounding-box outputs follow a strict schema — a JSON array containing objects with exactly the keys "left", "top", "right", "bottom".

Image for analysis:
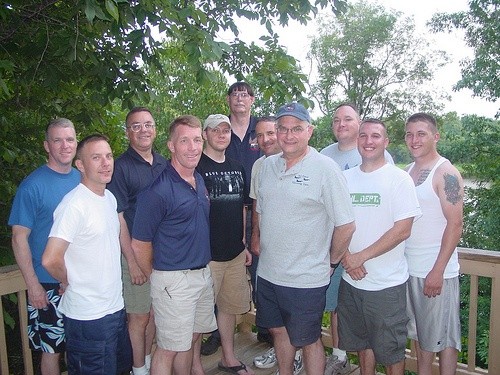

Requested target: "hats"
[
  {"left": 203, "top": 114, "right": 231, "bottom": 131},
  {"left": 276, "top": 103, "right": 311, "bottom": 124}
]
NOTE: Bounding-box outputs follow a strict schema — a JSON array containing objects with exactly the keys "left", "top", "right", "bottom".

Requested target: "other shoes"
[
  {"left": 257, "top": 333, "right": 273, "bottom": 346},
  {"left": 200, "top": 335, "right": 221, "bottom": 355}
]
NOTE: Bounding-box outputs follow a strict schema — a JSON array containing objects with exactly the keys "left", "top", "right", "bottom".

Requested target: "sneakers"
[
  {"left": 253, "top": 346, "right": 278, "bottom": 369},
  {"left": 322, "top": 353, "right": 351, "bottom": 375},
  {"left": 273, "top": 349, "right": 305, "bottom": 375}
]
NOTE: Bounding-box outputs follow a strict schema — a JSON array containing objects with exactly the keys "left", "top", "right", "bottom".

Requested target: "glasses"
[
  {"left": 127, "top": 124, "right": 156, "bottom": 133},
  {"left": 229, "top": 93, "right": 253, "bottom": 100},
  {"left": 276, "top": 126, "right": 308, "bottom": 134}
]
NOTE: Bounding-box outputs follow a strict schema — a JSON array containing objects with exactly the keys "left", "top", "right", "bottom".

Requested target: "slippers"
[{"left": 217, "top": 360, "right": 248, "bottom": 375}]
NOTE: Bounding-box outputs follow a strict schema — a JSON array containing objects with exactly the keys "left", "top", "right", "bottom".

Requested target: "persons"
[
  {"left": 401, "top": 112, "right": 464, "bottom": 375},
  {"left": 318, "top": 102, "right": 395, "bottom": 375},
  {"left": 131, "top": 111, "right": 218, "bottom": 375},
  {"left": 42, "top": 133, "right": 135, "bottom": 375},
  {"left": 8, "top": 117, "right": 82, "bottom": 375},
  {"left": 105, "top": 106, "right": 158, "bottom": 375},
  {"left": 248, "top": 116, "right": 305, "bottom": 375},
  {"left": 338, "top": 118, "right": 426, "bottom": 375},
  {"left": 199, "top": 81, "right": 275, "bottom": 355},
  {"left": 255, "top": 100, "right": 356, "bottom": 375},
  {"left": 195, "top": 110, "right": 256, "bottom": 375}
]
